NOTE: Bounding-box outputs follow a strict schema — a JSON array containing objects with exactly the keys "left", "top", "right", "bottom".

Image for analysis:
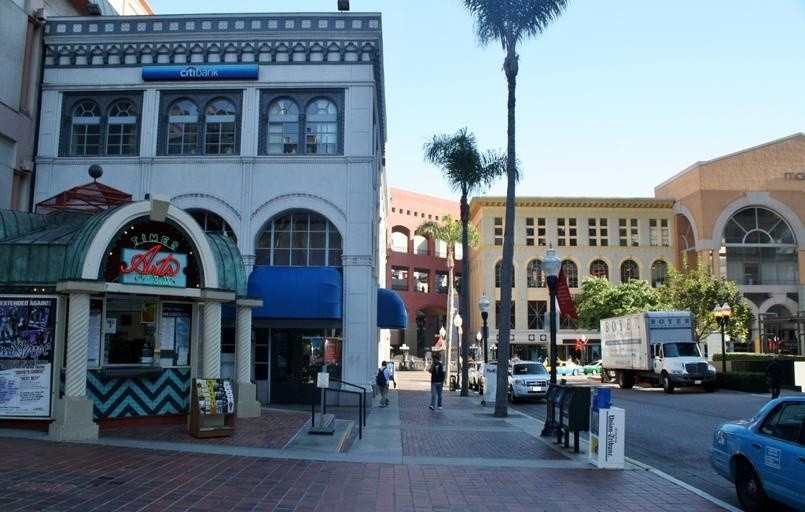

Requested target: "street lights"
[
  {"left": 439, "top": 325, "right": 447, "bottom": 373},
  {"left": 454, "top": 314, "right": 462, "bottom": 389},
  {"left": 489, "top": 343, "right": 497, "bottom": 359},
  {"left": 479, "top": 291, "right": 491, "bottom": 363},
  {"left": 580, "top": 333, "right": 588, "bottom": 366},
  {"left": 714, "top": 303, "right": 732, "bottom": 374},
  {"left": 476, "top": 332, "right": 482, "bottom": 360},
  {"left": 399, "top": 343, "right": 410, "bottom": 362},
  {"left": 541, "top": 241, "right": 565, "bottom": 436}
]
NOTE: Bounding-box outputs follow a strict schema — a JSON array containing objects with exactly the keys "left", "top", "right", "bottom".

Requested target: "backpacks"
[{"left": 376, "top": 368, "right": 387, "bottom": 387}]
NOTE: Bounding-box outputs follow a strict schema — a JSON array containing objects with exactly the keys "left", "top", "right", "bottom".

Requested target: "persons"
[
  {"left": 376, "top": 360, "right": 396, "bottom": 407},
  {"left": 427, "top": 355, "right": 446, "bottom": 411}
]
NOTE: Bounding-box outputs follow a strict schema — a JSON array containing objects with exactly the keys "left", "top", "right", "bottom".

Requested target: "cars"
[
  {"left": 707, "top": 394, "right": 805, "bottom": 512},
  {"left": 443, "top": 355, "right": 604, "bottom": 403}
]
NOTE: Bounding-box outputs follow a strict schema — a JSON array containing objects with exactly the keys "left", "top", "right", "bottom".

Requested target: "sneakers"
[{"left": 429, "top": 405, "right": 442, "bottom": 410}]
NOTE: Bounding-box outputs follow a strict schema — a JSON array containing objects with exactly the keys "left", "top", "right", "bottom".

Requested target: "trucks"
[{"left": 600, "top": 310, "right": 719, "bottom": 392}]
